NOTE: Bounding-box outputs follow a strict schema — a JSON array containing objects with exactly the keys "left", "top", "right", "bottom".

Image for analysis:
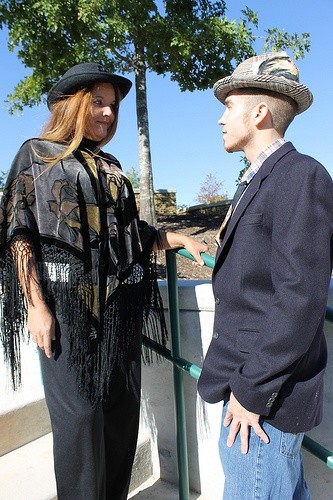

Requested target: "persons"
[
  {"left": 0, "top": 61, "right": 210, "bottom": 500},
  {"left": 193, "top": 50, "right": 333, "bottom": 500}
]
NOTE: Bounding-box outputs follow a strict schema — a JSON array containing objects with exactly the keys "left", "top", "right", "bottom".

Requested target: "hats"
[
  {"left": 47, "top": 62, "right": 133, "bottom": 113},
  {"left": 212, "top": 51, "right": 313, "bottom": 113}
]
